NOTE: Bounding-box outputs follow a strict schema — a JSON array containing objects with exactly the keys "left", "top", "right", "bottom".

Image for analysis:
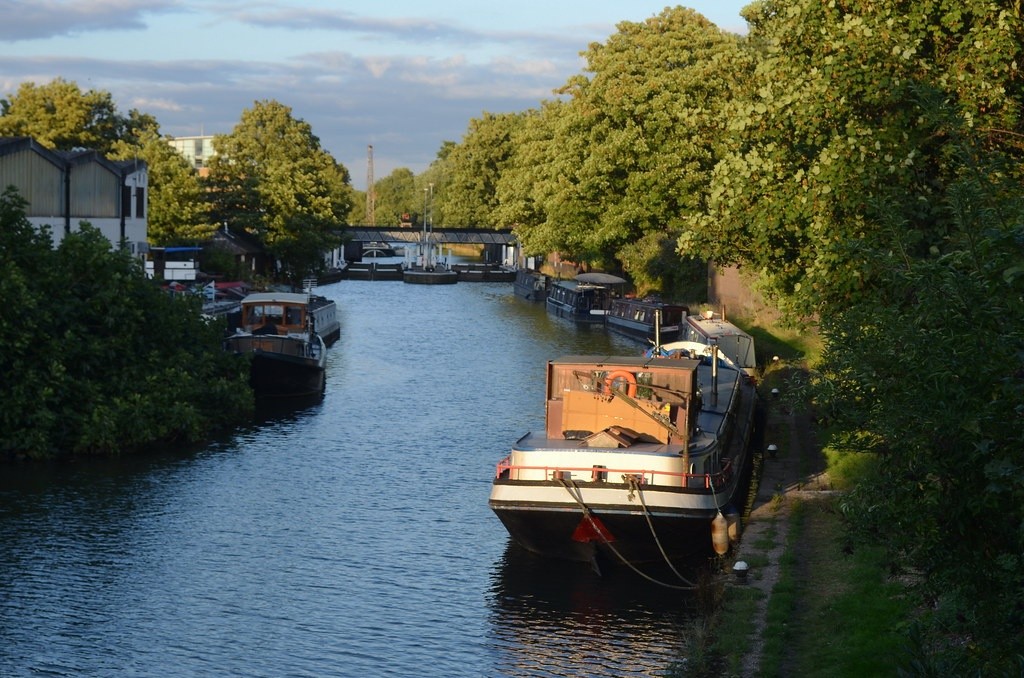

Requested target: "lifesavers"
[{"left": 605, "top": 369, "right": 637, "bottom": 401}]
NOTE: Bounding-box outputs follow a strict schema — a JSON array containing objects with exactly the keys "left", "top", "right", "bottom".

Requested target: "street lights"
[
  {"left": 424, "top": 188, "right": 428, "bottom": 247},
  {"left": 430, "top": 183, "right": 434, "bottom": 268}
]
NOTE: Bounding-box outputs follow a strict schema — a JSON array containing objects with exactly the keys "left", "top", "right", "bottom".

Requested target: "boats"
[
  {"left": 605, "top": 299, "right": 691, "bottom": 346},
  {"left": 513, "top": 269, "right": 553, "bottom": 301},
  {"left": 546, "top": 273, "right": 627, "bottom": 324},
  {"left": 488, "top": 310, "right": 759, "bottom": 564},
  {"left": 242, "top": 278, "right": 341, "bottom": 348},
  {"left": 678, "top": 304, "right": 756, "bottom": 381},
  {"left": 221, "top": 323, "right": 326, "bottom": 401}
]
